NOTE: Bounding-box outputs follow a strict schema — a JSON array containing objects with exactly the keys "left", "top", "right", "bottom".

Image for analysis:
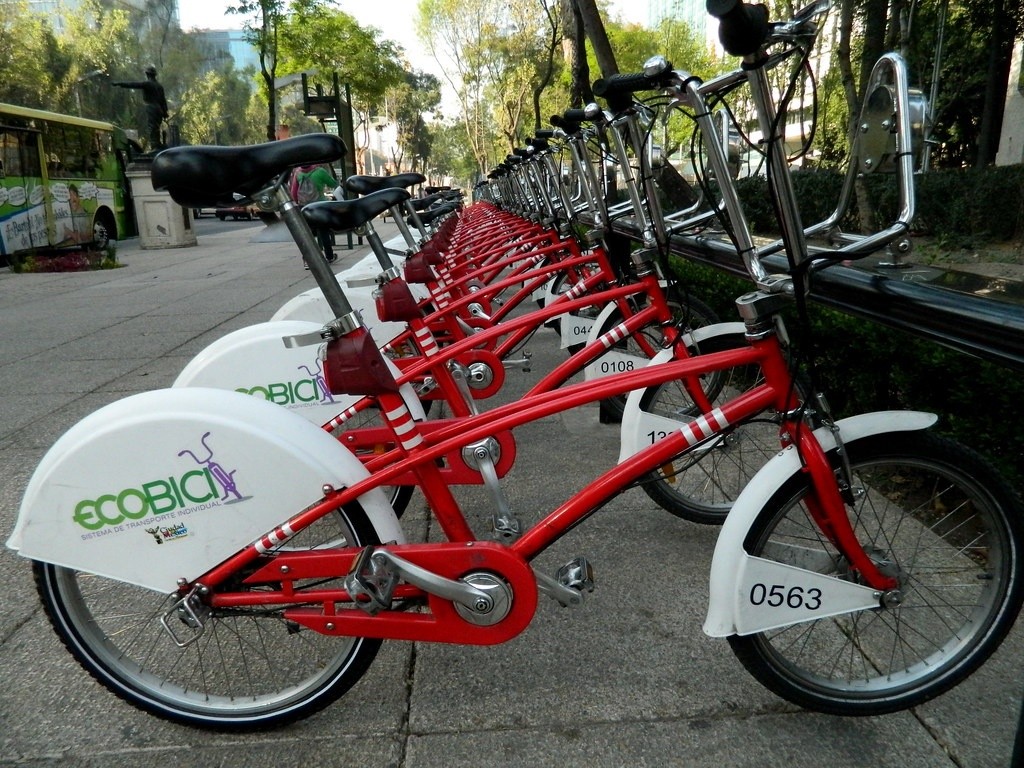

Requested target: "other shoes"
[
  {"left": 304, "top": 259, "right": 309, "bottom": 269},
  {"left": 325, "top": 253, "right": 338, "bottom": 264}
]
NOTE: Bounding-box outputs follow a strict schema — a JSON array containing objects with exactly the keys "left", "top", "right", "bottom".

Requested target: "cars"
[
  {"left": 216, "top": 202, "right": 261, "bottom": 222},
  {"left": 192, "top": 207, "right": 217, "bottom": 219}
]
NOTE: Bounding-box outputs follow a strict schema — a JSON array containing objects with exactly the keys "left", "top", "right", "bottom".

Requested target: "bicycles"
[
  {"left": 3, "top": 1, "right": 1024, "bottom": 738},
  {"left": 170, "top": 48, "right": 819, "bottom": 562}
]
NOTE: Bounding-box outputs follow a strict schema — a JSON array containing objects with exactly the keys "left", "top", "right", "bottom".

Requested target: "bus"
[{"left": 0, "top": 103, "right": 144, "bottom": 260}]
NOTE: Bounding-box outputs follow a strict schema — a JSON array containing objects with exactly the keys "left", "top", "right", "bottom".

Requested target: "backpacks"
[{"left": 296, "top": 168, "right": 320, "bottom": 206}]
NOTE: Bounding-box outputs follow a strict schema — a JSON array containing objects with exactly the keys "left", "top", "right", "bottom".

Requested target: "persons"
[
  {"left": 290, "top": 163, "right": 338, "bottom": 269},
  {"left": 111, "top": 68, "right": 169, "bottom": 151},
  {"left": 386, "top": 169, "right": 390, "bottom": 176}
]
